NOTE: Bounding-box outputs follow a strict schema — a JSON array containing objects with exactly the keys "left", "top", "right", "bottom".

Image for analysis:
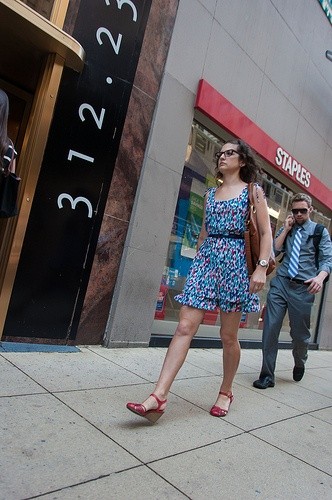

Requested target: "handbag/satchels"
[
  {"left": 244, "top": 181, "right": 276, "bottom": 275},
  {"left": 0, "top": 138, "right": 22, "bottom": 218}
]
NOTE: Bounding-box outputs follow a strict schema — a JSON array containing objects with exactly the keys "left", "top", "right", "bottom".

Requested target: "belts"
[{"left": 281, "top": 276, "right": 309, "bottom": 285}]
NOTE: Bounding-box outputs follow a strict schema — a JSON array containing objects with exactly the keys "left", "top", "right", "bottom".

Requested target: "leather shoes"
[
  {"left": 293, "top": 366, "right": 305, "bottom": 381},
  {"left": 253, "top": 377, "right": 275, "bottom": 389}
]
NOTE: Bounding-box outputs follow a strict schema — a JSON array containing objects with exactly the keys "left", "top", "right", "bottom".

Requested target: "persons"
[
  {"left": 254, "top": 191, "right": 332, "bottom": 390},
  {"left": 125, "top": 138, "right": 272, "bottom": 421},
  {"left": 0, "top": 90, "right": 18, "bottom": 175}
]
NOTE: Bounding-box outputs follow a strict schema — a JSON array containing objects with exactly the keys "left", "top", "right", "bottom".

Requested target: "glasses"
[
  {"left": 291, "top": 208, "right": 308, "bottom": 215},
  {"left": 215, "top": 149, "right": 242, "bottom": 159}
]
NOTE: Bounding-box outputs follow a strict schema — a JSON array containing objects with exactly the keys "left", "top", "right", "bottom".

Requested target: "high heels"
[
  {"left": 126, "top": 394, "right": 168, "bottom": 423},
  {"left": 210, "top": 391, "right": 234, "bottom": 418}
]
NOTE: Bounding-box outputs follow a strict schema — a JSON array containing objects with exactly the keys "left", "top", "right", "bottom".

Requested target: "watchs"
[{"left": 255, "top": 259, "right": 269, "bottom": 269}]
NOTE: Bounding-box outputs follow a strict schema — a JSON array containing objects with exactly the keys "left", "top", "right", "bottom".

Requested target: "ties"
[{"left": 288, "top": 226, "right": 303, "bottom": 280}]
